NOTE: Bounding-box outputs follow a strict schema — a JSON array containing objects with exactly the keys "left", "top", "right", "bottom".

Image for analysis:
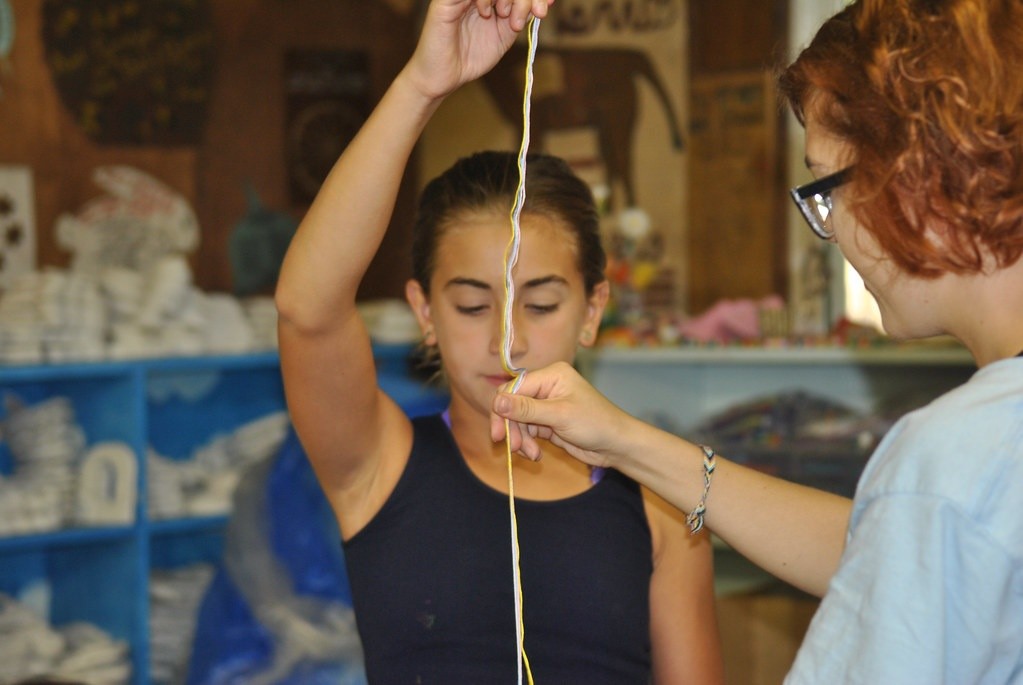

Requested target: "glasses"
[{"left": 790, "top": 164, "right": 855, "bottom": 239}]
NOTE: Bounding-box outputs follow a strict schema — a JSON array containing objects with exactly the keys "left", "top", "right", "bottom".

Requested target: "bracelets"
[{"left": 687, "top": 443, "right": 716, "bottom": 534}]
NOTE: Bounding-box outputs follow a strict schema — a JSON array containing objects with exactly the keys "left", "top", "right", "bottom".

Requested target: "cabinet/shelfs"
[{"left": 0, "top": 338, "right": 442, "bottom": 685}]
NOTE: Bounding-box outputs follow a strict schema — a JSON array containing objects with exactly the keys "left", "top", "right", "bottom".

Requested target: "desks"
[{"left": 574, "top": 342, "right": 978, "bottom": 431}]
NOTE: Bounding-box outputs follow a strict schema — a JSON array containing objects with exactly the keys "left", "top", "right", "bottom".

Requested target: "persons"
[
  {"left": 274, "top": 0, "right": 727, "bottom": 685},
  {"left": 488, "top": 0, "right": 1022, "bottom": 685}
]
